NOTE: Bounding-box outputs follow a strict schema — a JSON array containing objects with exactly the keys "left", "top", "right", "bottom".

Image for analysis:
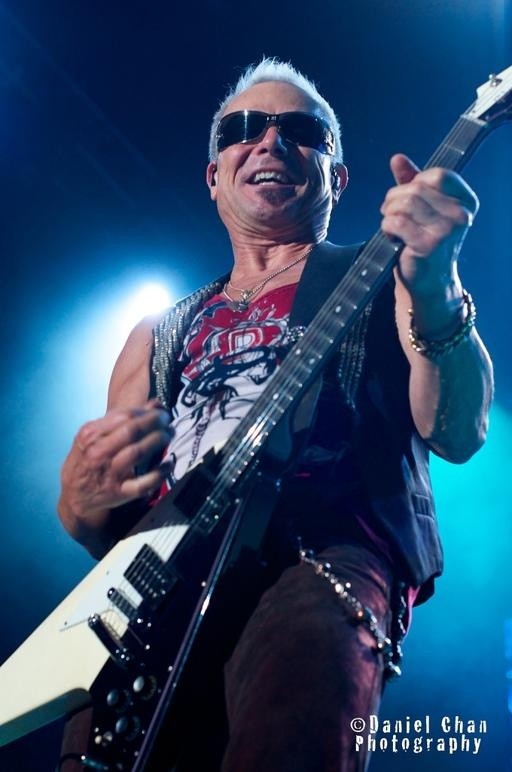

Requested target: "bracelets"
[{"left": 406, "top": 286, "right": 479, "bottom": 354}]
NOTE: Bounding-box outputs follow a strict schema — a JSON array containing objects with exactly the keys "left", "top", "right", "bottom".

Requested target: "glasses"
[{"left": 217, "top": 108, "right": 337, "bottom": 157}]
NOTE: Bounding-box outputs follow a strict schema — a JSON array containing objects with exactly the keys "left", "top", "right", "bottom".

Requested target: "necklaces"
[
  {"left": 222, "top": 274, "right": 280, "bottom": 312},
  {"left": 228, "top": 243, "right": 315, "bottom": 299}
]
